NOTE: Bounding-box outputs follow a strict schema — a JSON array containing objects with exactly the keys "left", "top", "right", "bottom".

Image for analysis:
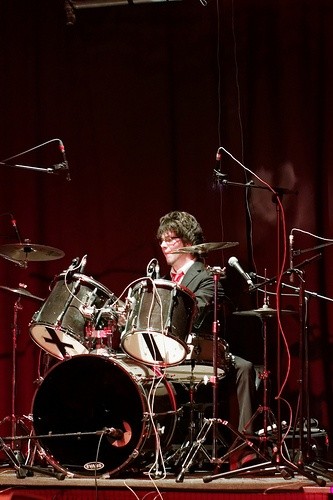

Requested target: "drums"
[
  {"left": 120, "top": 280, "right": 198, "bottom": 368},
  {"left": 31, "top": 353, "right": 177, "bottom": 479},
  {"left": 27, "top": 270, "right": 119, "bottom": 360},
  {"left": 164, "top": 334, "right": 229, "bottom": 383}
]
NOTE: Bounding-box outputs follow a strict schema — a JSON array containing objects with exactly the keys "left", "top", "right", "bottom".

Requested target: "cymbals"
[
  {"left": 233, "top": 308, "right": 296, "bottom": 316},
  {"left": 0, "top": 286, "right": 45, "bottom": 301},
  {"left": 0, "top": 244, "right": 65, "bottom": 262},
  {"left": 166, "top": 241, "right": 240, "bottom": 255}
]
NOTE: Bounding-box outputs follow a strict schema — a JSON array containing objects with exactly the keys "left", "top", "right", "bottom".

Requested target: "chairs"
[{"left": 229, "top": 310, "right": 266, "bottom": 393}]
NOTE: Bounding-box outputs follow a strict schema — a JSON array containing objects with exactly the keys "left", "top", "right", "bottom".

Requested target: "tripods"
[{"left": 0, "top": 148, "right": 333, "bottom": 487}]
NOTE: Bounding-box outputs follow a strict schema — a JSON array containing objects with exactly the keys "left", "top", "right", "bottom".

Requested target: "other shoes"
[{"left": 229, "top": 448, "right": 258, "bottom": 470}]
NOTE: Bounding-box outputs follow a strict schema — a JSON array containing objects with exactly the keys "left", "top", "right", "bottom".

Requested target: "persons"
[{"left": 157, "top": 211, "right": 257, "bottom": 439}]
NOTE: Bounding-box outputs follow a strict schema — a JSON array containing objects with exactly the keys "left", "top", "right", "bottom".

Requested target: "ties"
[{"left": 171, "top": 272, "right": 185, "bottom": 283}]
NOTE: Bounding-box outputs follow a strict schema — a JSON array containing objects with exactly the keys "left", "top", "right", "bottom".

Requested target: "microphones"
[
  {"left": 228, "top": 256, "right": 252, "bottom": 285},
  {"left": 213, "top": 153, "right": 221, "bottom": 183},
  {"left": 107, "top": 427, "right": 124, "bottom": 439},
  {"left": 80, "top": 257, "right": 87, "bottom": 273},
  {"left": 59, "top": 143, "right": 69, "bottom": 170}
]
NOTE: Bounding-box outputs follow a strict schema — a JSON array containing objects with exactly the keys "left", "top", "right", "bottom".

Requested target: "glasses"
[{"left": 158, "top": 236, "right": 183, "bottom": 244}]
